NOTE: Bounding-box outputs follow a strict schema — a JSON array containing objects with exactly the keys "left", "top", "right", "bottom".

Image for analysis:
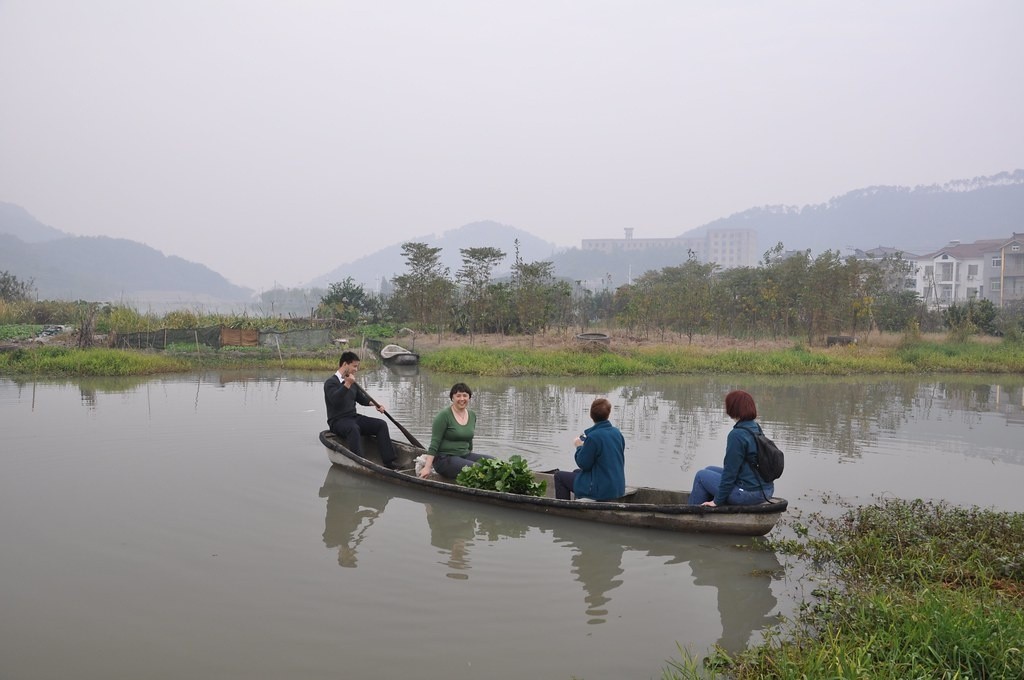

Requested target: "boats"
[
  {"left": 381, "top": 344, "right": 418, "bottom": 364},
  {"left": 319, "top": 428, "right": 789, "bottom": 538}
]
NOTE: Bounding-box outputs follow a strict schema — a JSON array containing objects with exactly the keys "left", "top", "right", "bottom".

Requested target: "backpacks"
[{"left": 735, "top": 426, "right": 785, "bottom": 483}]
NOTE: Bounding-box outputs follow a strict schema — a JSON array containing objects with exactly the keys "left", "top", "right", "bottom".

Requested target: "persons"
[
  {"left": 324, "top": 351, "right": 405, "bottom": 470},
  {"left": 687, "top": 390, "right": 775, "bottom": 507},
  {"left": 553, "top": 398, "right": 625, "bottom": 501},
  {"left": 418, "top": 383, "right": 496, "bottom": 479}
]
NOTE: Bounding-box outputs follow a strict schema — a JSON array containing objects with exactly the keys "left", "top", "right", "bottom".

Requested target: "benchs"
[
  {"left": 419, "top": 474, "right": 451, "bottom": 481},
  {"left": 574, "top": 489, "right": 638, "bottom": 501}
]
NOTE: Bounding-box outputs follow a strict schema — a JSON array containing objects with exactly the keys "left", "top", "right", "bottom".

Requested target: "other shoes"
[{"left": 385, "top": 461, "right": 405, "bottom": 470}]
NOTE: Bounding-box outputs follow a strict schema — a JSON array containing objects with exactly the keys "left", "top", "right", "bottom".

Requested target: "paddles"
[{"left": 343, "top": 373, "right": 425, "bottom": 448}]
